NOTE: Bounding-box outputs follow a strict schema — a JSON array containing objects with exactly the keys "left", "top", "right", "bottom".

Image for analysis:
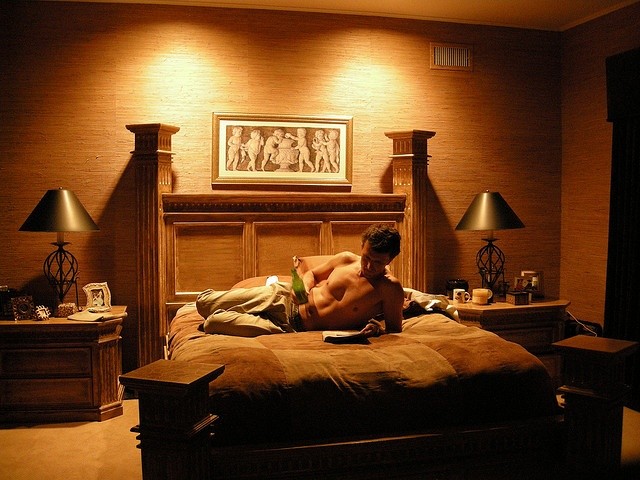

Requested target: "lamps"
[
  {"left": 17, "top": 186, "right": 102, "bottom": 312},
  {"left": 455, "top": 188, "right": 525, "bottom": 299}
]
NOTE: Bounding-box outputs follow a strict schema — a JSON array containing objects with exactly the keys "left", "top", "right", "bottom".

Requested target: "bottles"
[{"left": 291, "top": 268, "right": 310, "bottom": 305}]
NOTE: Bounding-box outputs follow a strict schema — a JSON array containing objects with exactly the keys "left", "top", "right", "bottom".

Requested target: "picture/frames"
[
  {"left": 82, "top": 282, "right": 112, "bottom": 311},
  {"left": 210, "top": 111, "right": 354, "bottom": 186}
]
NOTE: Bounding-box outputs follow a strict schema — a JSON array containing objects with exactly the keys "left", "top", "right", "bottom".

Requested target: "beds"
[{"left": 118, "top": 122, "right": 638, "bottom": 480}]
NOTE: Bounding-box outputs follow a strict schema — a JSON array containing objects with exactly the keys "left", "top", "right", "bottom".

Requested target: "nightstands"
[
  {"left": 0, "top": 304, "right": 129, "bottom": 421},
  {"left": 446, "top": 299, "right": 571, "bottom": 374}
]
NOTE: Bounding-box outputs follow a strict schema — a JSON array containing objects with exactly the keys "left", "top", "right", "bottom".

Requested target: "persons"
[
  {"left": 312, "top": 131, "right": 331, "bottom": 172},
  {"left": 261, "top": 129, "right": 285, "bottom": 170},
  {"left": 285, "top": 129, "right": 315, "bottom": 172},
  {"left": 243, "top": 130, "right": 264, "bottom": 170},
  {"left": 322, "top": 131, "right": 340, "bottom": 172},
  {"left": 196, "top": 222, "right": 405, "bottom": 338},
  {"left": 226, "top": 128, "right": 243, "bottom": 171}
]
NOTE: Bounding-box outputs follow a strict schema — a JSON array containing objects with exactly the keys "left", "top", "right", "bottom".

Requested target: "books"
[
  {"left": 67, "top": 310, "right": 128, "bottom": 322},
  {"left": 322, "top": 330, "right": 364, "bottom": 342}
]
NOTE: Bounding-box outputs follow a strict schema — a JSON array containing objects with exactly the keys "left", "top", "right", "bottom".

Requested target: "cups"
[
  {"left": 453, "top": 289, "right": 470, "bottom": 303},
  {"left": 471, "top": 289, "right": 493, "bottom": 305}
]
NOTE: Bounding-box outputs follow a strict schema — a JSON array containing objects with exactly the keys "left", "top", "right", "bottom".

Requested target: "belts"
[{"left": 290, "top": 302, "right": 304, "bottom": 332}]
